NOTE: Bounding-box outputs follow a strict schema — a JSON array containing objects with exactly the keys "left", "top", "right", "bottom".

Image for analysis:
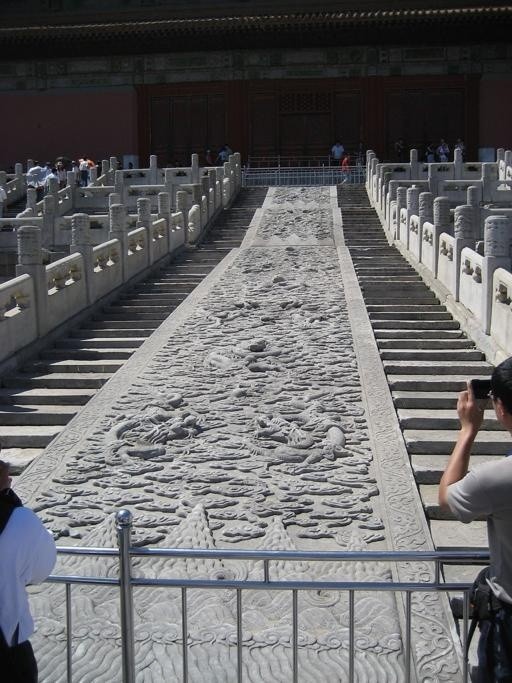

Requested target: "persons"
[
  {"left": 439, "top": 357, "right": 511, "bottom": 682},
  {"left": 328, "top": 137, "right": 466, "bottom": 186},
  {"left": 0, "top": 185, "right": 8, "bottom": 217},
  {"left": 25, "top": 157, "right": 97, "bottom": 201},
  {"left": 1, "top": 460, "right": 57, "bottom": 682},
  {"left": 202, "top": 144, "right": 231, "bottom": 166}
]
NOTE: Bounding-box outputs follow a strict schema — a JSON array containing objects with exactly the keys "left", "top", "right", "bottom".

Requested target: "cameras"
[{"left": 471, "top": 379, "right": 493, "bottom": 399}]
